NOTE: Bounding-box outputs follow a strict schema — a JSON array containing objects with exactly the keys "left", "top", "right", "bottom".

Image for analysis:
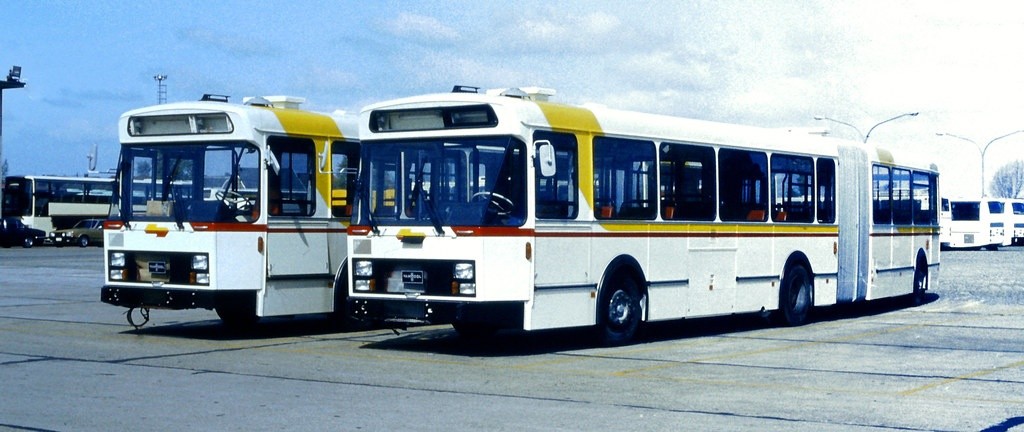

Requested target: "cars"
[{"left": 49, "top": 219, "right": 107, "bottom": 247}]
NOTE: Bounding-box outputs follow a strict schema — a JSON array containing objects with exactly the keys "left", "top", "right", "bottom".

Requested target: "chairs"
[
  {"left": 345, "top": 190, "right": 356, "bottom": 217},
  {"left": 250, "top": 180, "right": 284, "bottom": 222},
  {"left": 601, "top": 194, "right": 923, "bottom": 223}
]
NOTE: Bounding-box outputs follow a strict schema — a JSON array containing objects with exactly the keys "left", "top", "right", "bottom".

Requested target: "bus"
[
  {"left": 2, "top": 176, "right": 193, "bottom": 247},
  {"left": 346, "top": 85, "right": 941, "bottom": 346},
  {"left": 101, "top": 94, "right": 359, "bottom": 329},
  {"left": 941, "top": 199, "right": 1023, "bottom": 248}
]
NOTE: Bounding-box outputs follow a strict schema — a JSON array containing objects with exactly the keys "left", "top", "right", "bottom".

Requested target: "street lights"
[
  {"left": 936, "top": 129, "right": 1024, "bottom": 199},
  {"left": 154, "top": 75, "right": 168, "bottom": 103}
]
